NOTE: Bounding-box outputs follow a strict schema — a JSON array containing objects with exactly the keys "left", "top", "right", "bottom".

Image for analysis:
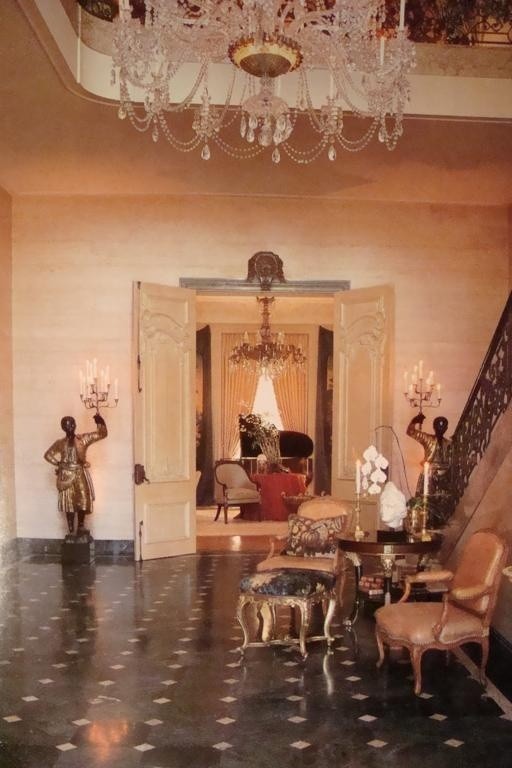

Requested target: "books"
[{"left": 357, "top": 570, "right": 427, "bottom": 600}]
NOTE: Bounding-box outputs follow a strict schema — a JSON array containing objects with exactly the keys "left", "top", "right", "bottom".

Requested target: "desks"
[
  {"left": 233, "top": 473, "right": 306, "bottom": 522},
  {"left": 335, "top": 530, "right": 445, "bottom": 608}
]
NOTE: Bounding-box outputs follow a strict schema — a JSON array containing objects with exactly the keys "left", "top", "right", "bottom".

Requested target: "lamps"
[
  {"left": 358, "top": 421, "right": 416, "bottom": 509},
  {"left": 227, "top": 295, "right": 309, "bottom": 377},
  {"left": 111, "top": 2, "right": 417, "bottom": 166}
]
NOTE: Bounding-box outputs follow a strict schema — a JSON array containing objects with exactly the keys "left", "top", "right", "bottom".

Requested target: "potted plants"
[
  {"left": 403, "top": 496, "right": 429, "bottom": 535},
  {"left": 249, "top": 414, "right": 282, "bottom": 473}
]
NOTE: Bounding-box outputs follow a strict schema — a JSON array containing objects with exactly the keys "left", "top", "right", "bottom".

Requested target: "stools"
[{"left": 236, "top": 568, "right": 338, "bottom": 661}]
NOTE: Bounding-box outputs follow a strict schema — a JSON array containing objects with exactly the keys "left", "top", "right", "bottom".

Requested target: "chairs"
[
  {"left": 373, "top": 528, "right": 509, "bottom": 696},
  {"left": 214, "top": 461, "right": 262, "bottom": 524},
  {"left": 256, "top": 495, "right": 354, "bottom": 609}
]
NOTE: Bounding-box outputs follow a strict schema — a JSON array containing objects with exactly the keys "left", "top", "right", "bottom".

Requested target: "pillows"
[{"left": 280, "top": 513, "right": 347, "bottom": 560}]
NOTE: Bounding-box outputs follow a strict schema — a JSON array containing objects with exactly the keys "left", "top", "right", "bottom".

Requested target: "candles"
[
  {"left": 403, "top": 360, "right": 442, "bottom": 403},
  {"left": 423, "top": 462, "right": 429, "bottom": 501},
  {"left": 80, "top": 359, "right": 120, "bottom": 402}
]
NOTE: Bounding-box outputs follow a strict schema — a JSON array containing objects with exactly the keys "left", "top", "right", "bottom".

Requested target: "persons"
[
  {"left": 44, "top": 411, "right": 107, "bottom": 544},
  {"left": 404, "top": 414, "right": 451, "bottom": 494}
]
